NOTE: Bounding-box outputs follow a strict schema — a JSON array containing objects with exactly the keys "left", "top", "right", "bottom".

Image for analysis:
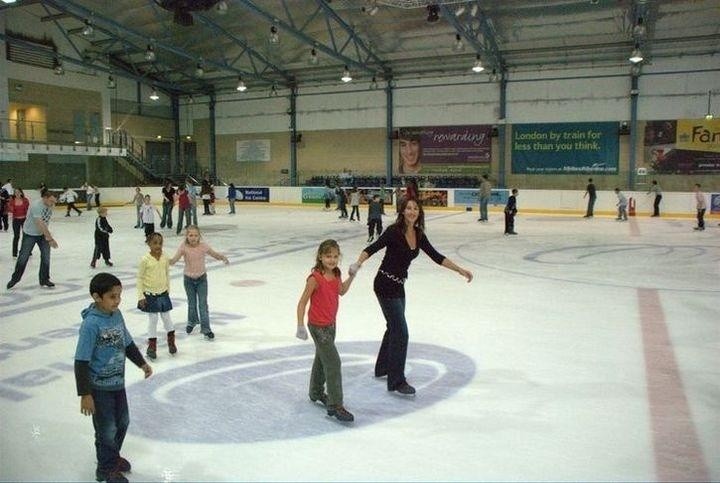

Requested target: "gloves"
[
  {"left": 348, "top": 262, "right": 361, "bottom": 275},
  {"left": 295, "top": 324, "right": 309, "bottom": 341}
]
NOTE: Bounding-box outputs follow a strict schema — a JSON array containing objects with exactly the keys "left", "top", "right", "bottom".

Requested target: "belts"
[{"left": 144, "top": 288, "right": 169, "bottom": 296}]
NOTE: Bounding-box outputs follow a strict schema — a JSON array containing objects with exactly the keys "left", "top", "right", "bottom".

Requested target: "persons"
[
  {"left": 504, "top": 188, "right": 519, "bottom": 236},
  {"left": 582, "top": 179, "right": 597, "bottom": 218},
  {"left": 349, "top": 202, "right": 474, "bottom": 392},
  {"left": 1, "top": 172, "right": 116, "bottom": 290},
  {"left": 125, "top": 176, "right": 239, "bottom": 238},
  {"left": 475, "top": 173, "right": 491, "bottom": 223},
  {"left": 646, "top": 180, "right": 665, "bottom": 217},
  {"left": 168, "top": 226, "right": 232, "bottom": 339},
  {"left": 399, "top": 132, "right": 424, "bottom": 175},
  {"left": 325, "top": 176, "right": 385, "bottom": 241},
  {"left": 296, "top": 239, "right": 356, "bottom": 425},
  {"left": 135, "top": 232, "right": 178, "bottom": 359},
  {"left": 690, "top": 184, "right": 708, "bottom": 231},
  {"left": 403, "top": 180, "right": 420, "bottom": 201},
  {"left": 71, "top": 273, "right": 152, "bottom": 483},
  {"left": 613, "top": 186, "right": 629, "bottom": 222}
]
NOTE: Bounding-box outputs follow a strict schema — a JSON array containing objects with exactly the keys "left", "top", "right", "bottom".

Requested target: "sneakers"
[
  {"left": 374, "top": 368, "right": 390, "bottom": 377},
  {"left": 91, "top": 260, "right": 96, "bottom": 267},
  {"left": 94, "top": 467, "right": 131, "bottom": 483},
  {"left": 504, "top": 231, "right": 519, "bottom": 236},
  {"left": 6, "top": 277, "right": 19, "bottom": 289},
  {"left": 39, "top": 278, "right": 55, "bottom": 287},
  {"left": 203, "top": 331, "right": 215, "bottom": 338},
  {"left": 387, "top": 378, "right": 417, "bottom": 394},
  {"left": 615, "top": 217, "right": 622, "bottom": 220},
  {"left": 114, "top": 455, "right": 132, "bottom": 472},
  {"left": 326, "top": 406, "right": 355, "bottom": 423},
  {"left": 478, "top": 218, "right": 488, "bottom": 221},
  {"left": 309, "top": 392, "right": 328, "bottom": 406},
  {"left": 105, "top": 260, "right": 113, "bottom": 266},
  {"left": 622, "top": 218, "right": 628, "bottom": 221},
  {"left": 367, "top": 235, "right": 374, "bottom": 242},
  {"left": 134, "top": 224, "right": 145, "bottom": 228},
  {"left": 186, "top": 325, "right": 194, "bottom": 333}
]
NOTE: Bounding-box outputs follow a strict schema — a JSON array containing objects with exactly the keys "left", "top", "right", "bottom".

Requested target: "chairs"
[{"left": 303, "top": 173, "right": 482, "bottom": 191}]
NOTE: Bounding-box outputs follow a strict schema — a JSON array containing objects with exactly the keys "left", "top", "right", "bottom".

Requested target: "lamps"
[
  {"left": 269, "top": 86, "right": 278, "bottom": 97},
  {"left": 309, "top": 47, "right": 319, "bottom": 65},
  {"left": 704, "top": 90, "right": 714, "bottom": 121},
  {"left": 107, "top": 75, "right": 117, "bottom": 89},
  {"left": 149, "top": 86, "right": 161, "bottom": 100},
  {"left": 235, "top": 72, "right": 247, "bottom": 92},
  {"left": 143, "top": 44, "right": 156, "bottom": 63},
  {"left": 425, "top": 2, "right": 507, "bottom": 84},
  {"left": 82, "top": 17, "right": 94, "bottom": 36},
  {"left": 341, "top": 65, "right": 352, "bottom": 83},
  {"left": 196, "top": 61, "right": 204, "bottom": 78},
  {"left": 268, "top": 25, "right": 280, "bottom": 44},
  {"left": 369, "top": 75, "right": 378, "bottom": 89},
  {"left": 54, "top": 64, "right": 65, "bottom": 76},
  {"left": 627, "top": 14, "right": 648, "bottom": 100},
  {"left": 361, "top": 4, "right": 381, "bottom": 16},
  {"left": 214, "top": 2, "right": 230, "bottom": 16}
]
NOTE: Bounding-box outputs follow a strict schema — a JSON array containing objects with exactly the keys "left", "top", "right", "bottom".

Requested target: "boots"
[
  {"left": 146, "top": 337, "right": 158, "bottom": 359},
  {"left": 166, "top": 329, "right": 178, "bottom": 353}
]
